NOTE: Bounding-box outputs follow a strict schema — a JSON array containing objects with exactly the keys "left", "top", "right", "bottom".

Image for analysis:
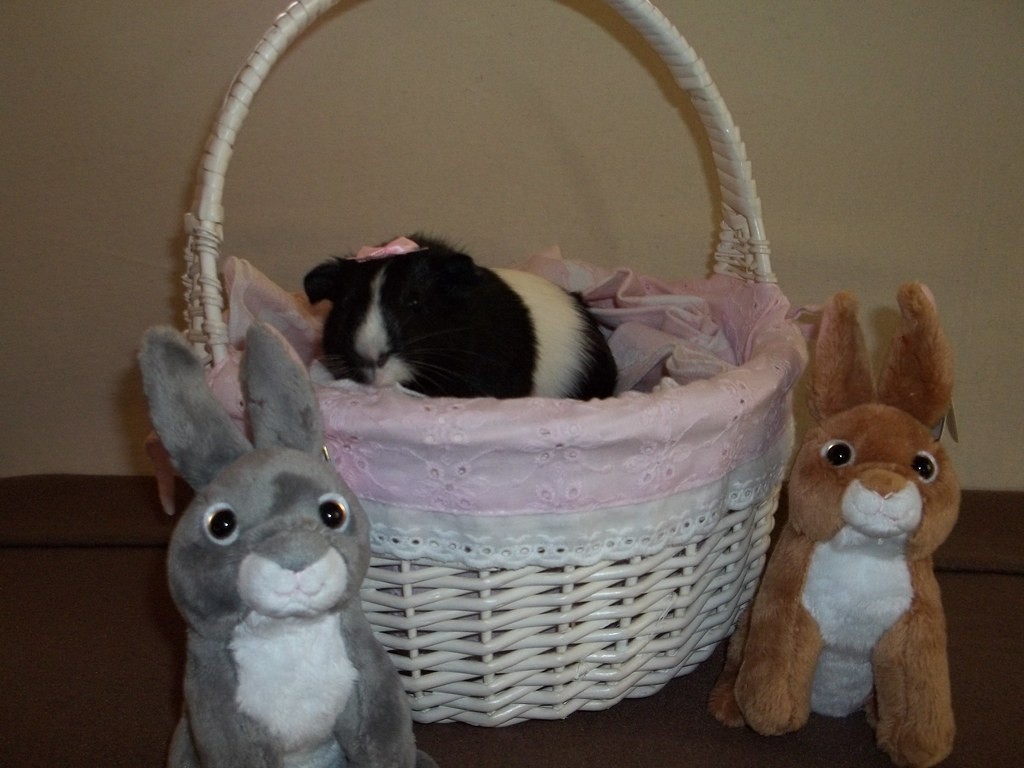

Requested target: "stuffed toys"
[
  {"left": 137, "top": 323, "right": 437, "bottom": 768},
  {"left": 710, "top": 281, "right": 957, "bottom": 768}
]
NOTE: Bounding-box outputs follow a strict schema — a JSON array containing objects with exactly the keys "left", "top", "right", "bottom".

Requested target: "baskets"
[{"left": 180, "top": 0, "right": 806, "bottom": 728}]
